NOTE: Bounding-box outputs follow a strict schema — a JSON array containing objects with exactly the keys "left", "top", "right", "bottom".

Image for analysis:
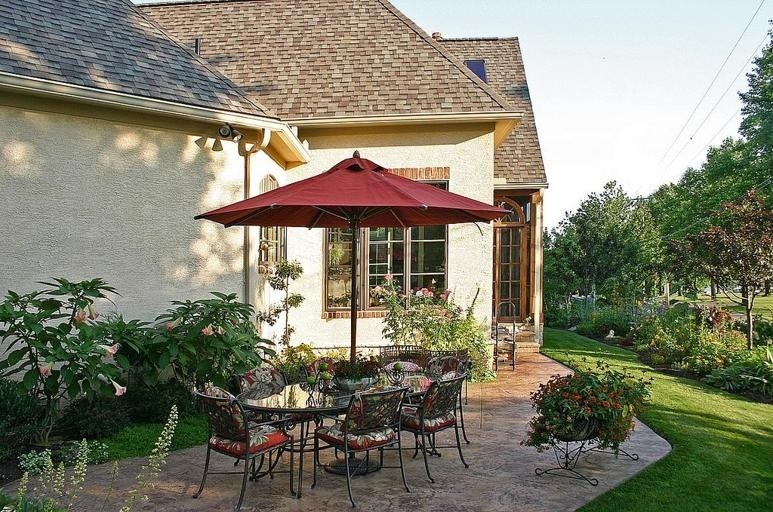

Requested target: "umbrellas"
[{"left": 191, "top": 148, "right": 511, "bottom": 461}]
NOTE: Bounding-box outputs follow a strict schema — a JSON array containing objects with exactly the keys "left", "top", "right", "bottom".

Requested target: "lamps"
[{"left": 218, "top": 124, "right": 244, "bottom": 142}]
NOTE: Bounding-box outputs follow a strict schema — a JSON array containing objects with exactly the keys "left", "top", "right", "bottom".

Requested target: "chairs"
[
  {"left": 302, "top": 356, "right": 359, "bottom": 464},
  {"left": 236, "top": 367, "right": 307, "bottom": 483},
  {"left": 378, "top": 375, "right": 468, "bottom": 484},
  {"left": 311, "top": 385, "right": 412, "bottom": 505},
  {"left": 406, "top": 355, "right": 470, "bottom": 455},
  {"left": 192, "top": 386, "right": 295, "bottom": 508}
]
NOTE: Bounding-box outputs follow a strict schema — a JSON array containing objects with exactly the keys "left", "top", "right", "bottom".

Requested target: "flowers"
[
  {"left": 522, "top": 365, "right": 653, "bottom": 456},
  {"left": 307, "top": 356, "right": 336, "bottom": 384}
]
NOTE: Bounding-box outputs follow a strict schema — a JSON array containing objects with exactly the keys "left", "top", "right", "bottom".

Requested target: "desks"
[{"left": 242, "top": 374, "right": 440, "bottom": 498}]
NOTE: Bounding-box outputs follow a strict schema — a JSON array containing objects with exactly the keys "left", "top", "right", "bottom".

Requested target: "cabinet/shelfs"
[{"left": 535, "top": 416, "right": 639, "bottom": 484}]
